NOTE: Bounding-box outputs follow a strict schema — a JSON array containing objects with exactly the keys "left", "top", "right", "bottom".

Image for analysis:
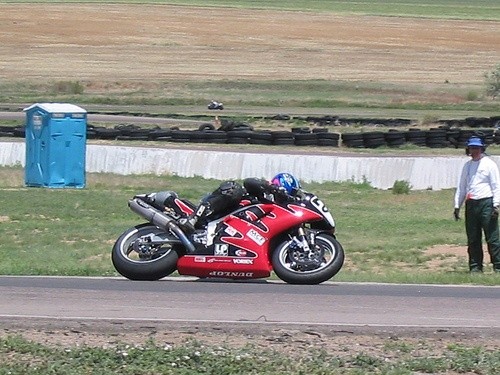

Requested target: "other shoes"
[{"left": 182, "top": 214, "right": 199, "bottom": 230}]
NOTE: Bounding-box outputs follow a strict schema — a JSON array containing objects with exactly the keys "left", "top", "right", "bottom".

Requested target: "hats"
[{"left": 467, "top": 136, "right": 485, "bottom": 146}]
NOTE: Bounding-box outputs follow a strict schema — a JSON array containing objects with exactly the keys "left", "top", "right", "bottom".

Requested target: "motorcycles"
[
  {"left": 207, "top": 103, "right": 224, "bottom": 110},
  {"left": 110, "top": 180, "right": 345, "bottom": 285}
]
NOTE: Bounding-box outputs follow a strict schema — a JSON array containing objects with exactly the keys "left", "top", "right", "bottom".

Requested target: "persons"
[
  {"left": 176, "top": 172, "right": 301, "bottom": 232},
  {"left": 211, "top": 100, "right": 217, "bottom": 109},
  {"left": 454, "top": 136, "right": 500, "bottom": 273}
]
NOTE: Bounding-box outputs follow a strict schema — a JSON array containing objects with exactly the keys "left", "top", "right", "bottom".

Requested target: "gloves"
[
  {"left": 453, "top": 209, "right": 461, "bottom": 222},
  {"left": 490, "top": 207, "right": 499, "bottom": 220}
]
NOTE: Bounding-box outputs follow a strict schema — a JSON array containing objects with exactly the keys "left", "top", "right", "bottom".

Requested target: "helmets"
[{"left": 271, "top": 173, "right": 300, "bottom": 194}]
